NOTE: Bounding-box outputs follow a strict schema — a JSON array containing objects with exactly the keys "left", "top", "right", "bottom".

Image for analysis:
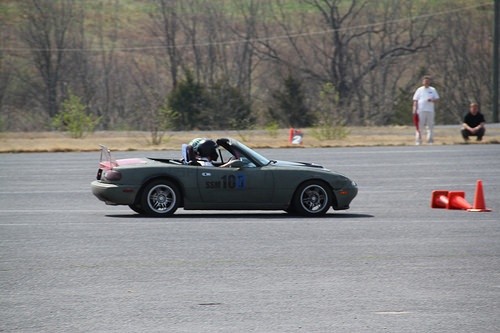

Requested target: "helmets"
[{"left": 196, "top": 139, "right": 219, "bottom": 161}]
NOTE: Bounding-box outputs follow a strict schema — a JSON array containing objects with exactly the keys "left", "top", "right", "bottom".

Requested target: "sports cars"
[{"left": 87, "top": 135, "right": 358, "bottom": 218}]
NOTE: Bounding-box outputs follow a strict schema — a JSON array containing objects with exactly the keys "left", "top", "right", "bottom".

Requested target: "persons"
[
  {"left": 189, "top": 138, "right": 204, "bottom": 159},
  {"left": 411, "top": 76, "right": 441, "bottom": 146},
  {"left": 460, "top": 101, "right": 486, "bottom": 143},
  {"left": 195, "top": 138, "right": 240, "bottom": 170}
]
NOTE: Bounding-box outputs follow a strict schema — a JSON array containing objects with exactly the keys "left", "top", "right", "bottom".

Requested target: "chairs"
[{"left": 183, "top": 144, "right": 201, "bottom": 167}]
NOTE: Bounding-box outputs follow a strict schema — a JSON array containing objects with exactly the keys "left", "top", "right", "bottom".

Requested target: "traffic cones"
[
  {"left": 466, "top": 180, "right": 492, "bottom": 212},
  {"left": 430, "top": 190, "right": 450, "bottom": 209},
  {"left": 446, "top": 190, "right": 473, "bottom": 211}
]
